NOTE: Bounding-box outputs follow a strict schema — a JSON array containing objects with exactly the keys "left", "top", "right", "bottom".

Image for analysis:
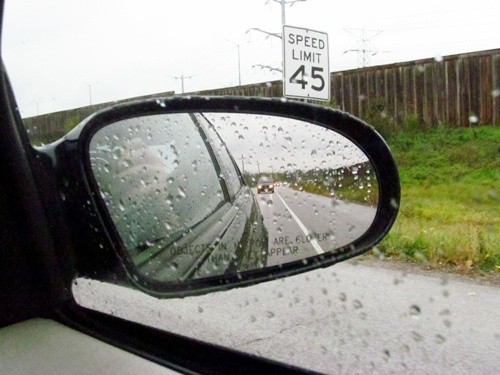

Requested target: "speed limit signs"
[{"left": 282, "top": 25, "right": 331, "bottom": 102}]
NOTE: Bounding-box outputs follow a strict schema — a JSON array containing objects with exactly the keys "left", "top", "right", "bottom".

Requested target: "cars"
[
  {"left": 88, "top": 108, "right": 270, "bottom": 280},
  {"left": 257, "top": 173, "right": 274, "bottom": 193}
]
